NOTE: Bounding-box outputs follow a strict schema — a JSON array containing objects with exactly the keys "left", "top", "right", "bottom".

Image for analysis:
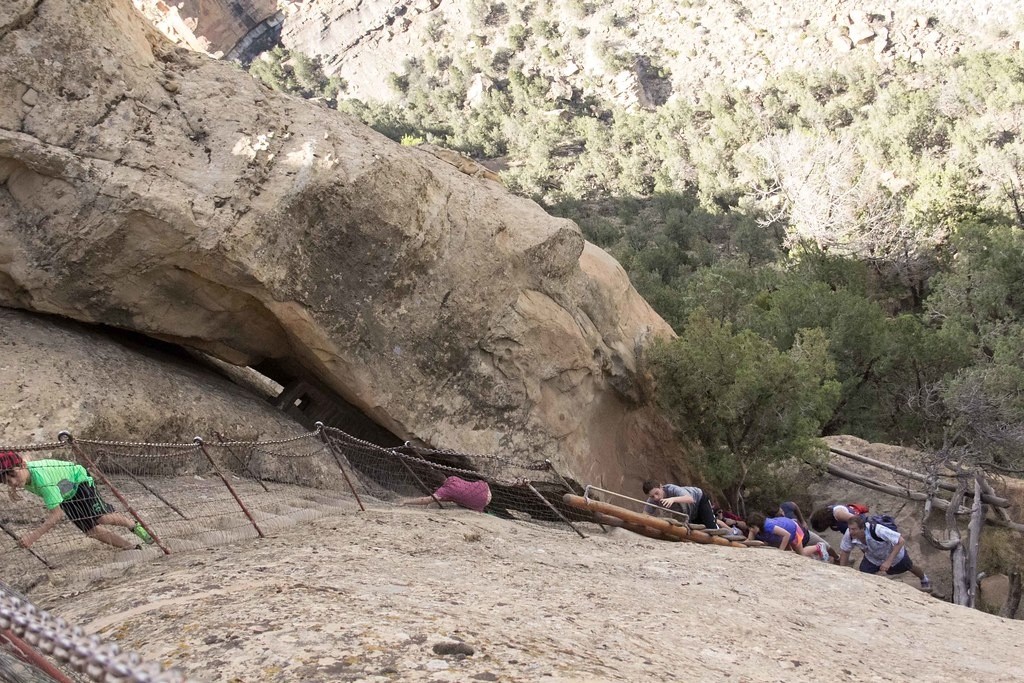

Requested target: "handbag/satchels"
[{"left": 848, "top": 503, "right": 870, "bottom": 513}]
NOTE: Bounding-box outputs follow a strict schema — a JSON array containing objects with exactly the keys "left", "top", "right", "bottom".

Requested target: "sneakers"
[
  {"left": 132, "top": 520, "right": 156, "bottom": 544},
  {"left": 921, "top": 580, "right": 932, "bottom": 592},
  {"left": 817, "top": 542, "right": 829, "bottom": 561}
]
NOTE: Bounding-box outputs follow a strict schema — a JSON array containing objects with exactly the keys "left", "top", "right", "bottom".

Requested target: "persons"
[
  {"left": 710, "top": 504, "right": 748, "bottom": 535},
  {"left": 744, "top": 514, "right": 830, "bottom": 562},
  {"left": 394, "top": 465, "right": 554, "bottom": 520},
  {"left": 640, "top": 479, "right": 743, "bottom": 541},
  {"left": 838, "top": 516, "right": 930, "bottom": 592},
  {"left": 0, "top": 447, "right": 156, "bottom": 554},
  {"left": 808, "top": 503, "right": 870, "bottom": 536},
  {"left": 763, "top": 500, "right": 842, "bottom": 565}
]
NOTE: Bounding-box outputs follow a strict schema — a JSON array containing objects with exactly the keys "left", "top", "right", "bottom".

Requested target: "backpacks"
[{"left": 849, "top": 515, "right": 897, "bottom": 542}]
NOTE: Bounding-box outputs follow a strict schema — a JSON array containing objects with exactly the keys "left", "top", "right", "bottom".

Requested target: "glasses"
[{"left": 715, "top": 510, "right": 723, "bottom": 517}]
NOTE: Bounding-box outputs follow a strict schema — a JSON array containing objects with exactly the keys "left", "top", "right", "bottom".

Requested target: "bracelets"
[{"left": 735, "top": 521, "right": 739, "bottom": 525}]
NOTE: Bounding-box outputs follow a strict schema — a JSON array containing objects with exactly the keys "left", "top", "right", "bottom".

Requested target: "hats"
[
  {"left": 1, "top": 452, "right": 21, "bottom": 483},
  {"left": 711, "top": 504, "right": 721, "bottom": 513}
]
